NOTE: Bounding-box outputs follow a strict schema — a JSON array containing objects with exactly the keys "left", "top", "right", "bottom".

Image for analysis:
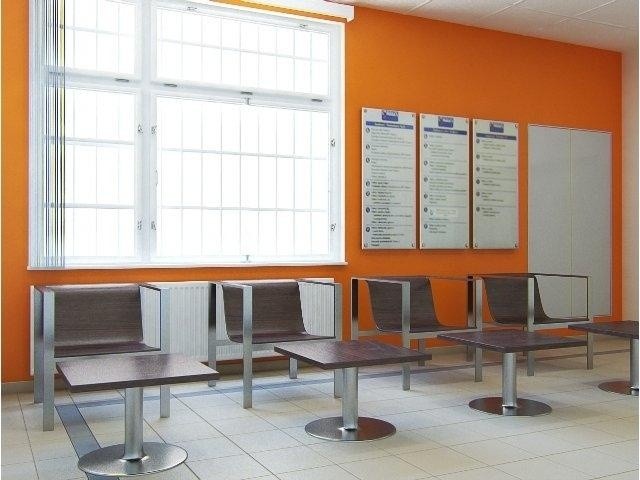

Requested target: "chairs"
[
  {"left": 349, "top": 274, "right": 483, "bottom": 390},
  {"left": 466, "top": 273, "right": 593, "bottom": 383},
  {"left": 33, "top": 282, "right": 171, "bottom": 430},
  {"left": 208, "top": 280, "right": 344, "bottom": 409}
]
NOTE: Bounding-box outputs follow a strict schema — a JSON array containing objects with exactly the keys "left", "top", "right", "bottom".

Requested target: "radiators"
[{"left": 29, "top": 276, "right": 335, "bottom": 377}]
